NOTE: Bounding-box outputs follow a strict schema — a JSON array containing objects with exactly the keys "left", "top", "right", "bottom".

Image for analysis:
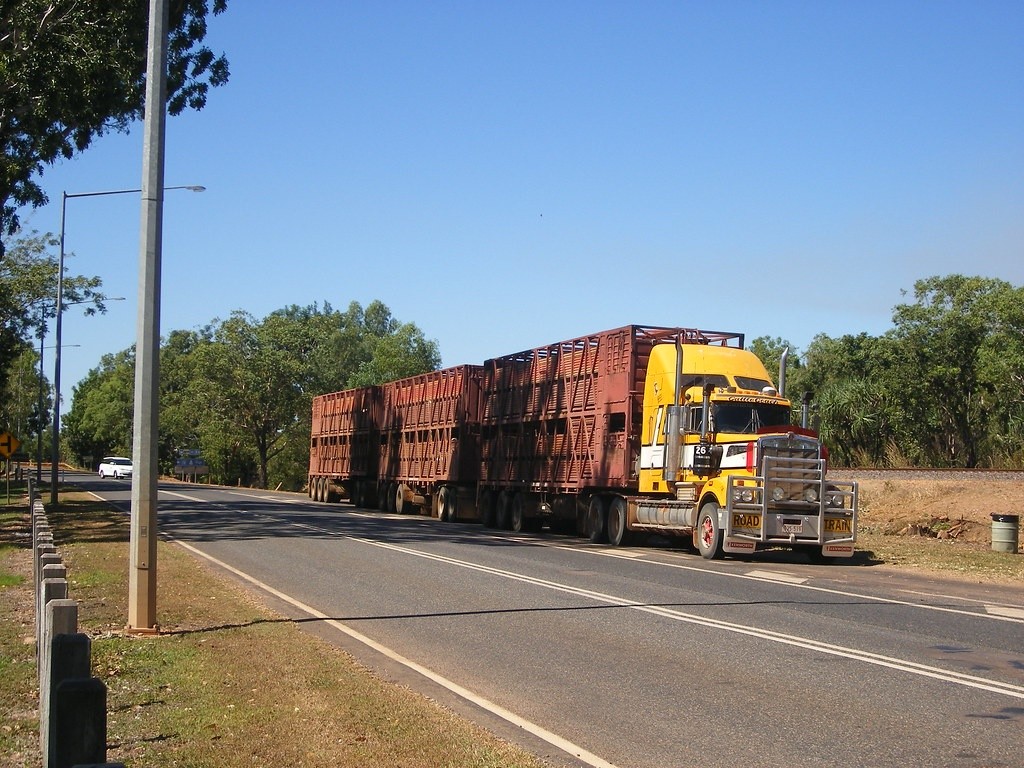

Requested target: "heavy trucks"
[{"left": 308, "top": 325, "right": 858, "bottom": 561}]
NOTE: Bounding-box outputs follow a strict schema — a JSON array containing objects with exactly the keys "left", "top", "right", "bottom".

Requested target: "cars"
[{"left": 98, "top": 457, "right": 133, "bottom": 479}]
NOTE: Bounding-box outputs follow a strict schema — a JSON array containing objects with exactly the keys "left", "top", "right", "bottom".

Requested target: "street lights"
[
  {"left": 37, "top": 296, "right": 126, "bottom": 485},
  {"left": 51, "top": 184, "right": 205, "bottom": 504},
  {"left": 18, "top": 345, "right": 81, "bottom": 475}
]
